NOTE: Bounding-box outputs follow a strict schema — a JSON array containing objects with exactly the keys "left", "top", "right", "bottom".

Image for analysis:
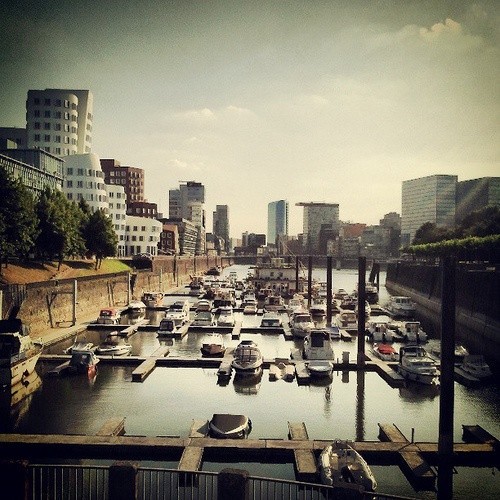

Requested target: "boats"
[
  {"left": 200, "top": 333, "right": 228, "bottom": 357},
  {"left": 303, "top": 330, "right": 335, "bottom": 360},
  {"left": 372, "top": 344, "right": 400, "bottom": 362},
  {"left": 10, "top": 370, "right": 44, "bottom": 407},
  {"left": 307, "top": 360, "right": 334, "bottom": 376},
  {"left": 232, "top": 340, "right": 263, "bottom": 374},
  {"left": 69, "top": 343, "right": 100, "bottom": 377},
  {"left": 97, "top": 234, "right": 429, "bottom": 343},
  {"left": 270, "top": 357, "right": 295, "bottom": 380},
  {"left": 0, "top": 318, "right": 45, "bottom": 386},
  {"left": 319, "top": 438, "right": 378, "bottom": 500},
  {"left": 397, "top": 346, "right": 437, "bottom": 385},
  {"left": 97, "top": 330, "right": 133, "bottom": 356},
  {"left": 209, "top": 414, "right": 251, "bottom": 439},
  {"left": 455, "top": 346, "right": 492, "bottom": 379}
]
